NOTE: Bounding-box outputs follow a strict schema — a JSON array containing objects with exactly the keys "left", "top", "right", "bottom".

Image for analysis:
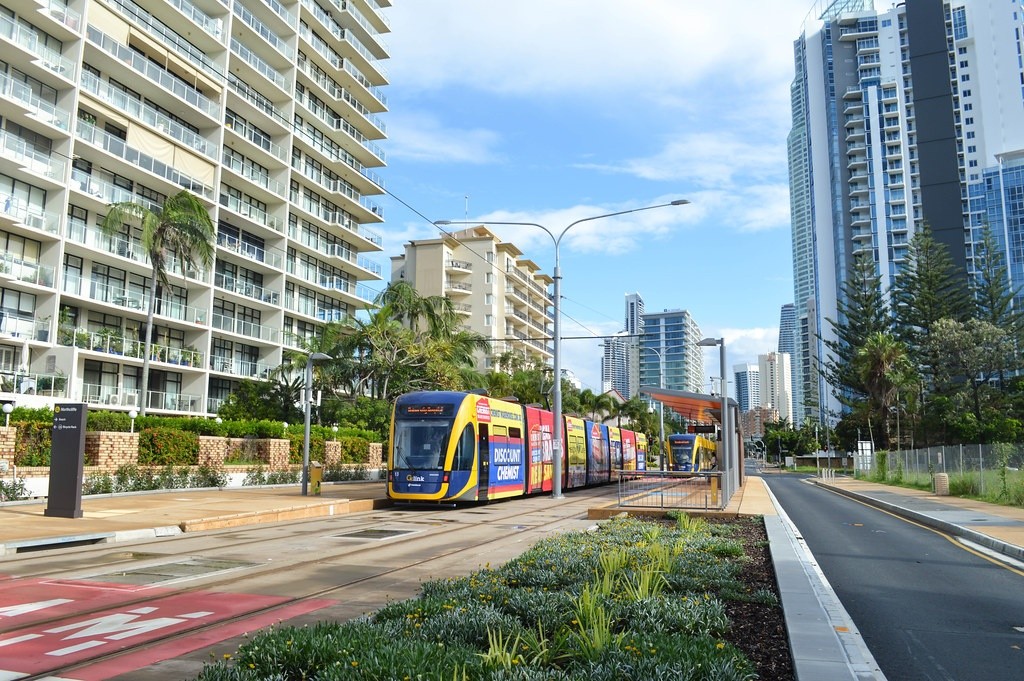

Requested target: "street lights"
[
  {"left": 598, "top": 343, "right": 697, "bottom": 472},
  {"left": 434, "top": 198, "right": 692, "bottom": 500},
  {"left": 301, "top": 352, "right": 333, "bottom": 494},
  {"left": 698, "top": 338, "right": 729, "bottom": 501}
]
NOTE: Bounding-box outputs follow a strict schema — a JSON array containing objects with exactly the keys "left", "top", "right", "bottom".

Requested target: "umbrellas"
[{"left": 19, "top": 338, "right": 29, "bottom": 372}]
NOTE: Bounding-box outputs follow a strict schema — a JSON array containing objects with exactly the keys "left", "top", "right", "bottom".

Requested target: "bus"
[
  {"left": 665, "top": 433, "right": 717, "bottom": 474},
  {"left": 383, "top": 390, "right": 647, "bottom": 508}
]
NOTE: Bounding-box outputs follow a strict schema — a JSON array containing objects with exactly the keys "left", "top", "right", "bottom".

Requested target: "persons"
[
  {"left": 710, "top": 452, "right": 717, "bottom": 469},
  {"left": 680, "top": 452, "right": 689, "bottom": 459}
]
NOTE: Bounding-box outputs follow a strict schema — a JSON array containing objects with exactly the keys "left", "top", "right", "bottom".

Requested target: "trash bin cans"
[
  {"left": 310, "top": 461, "right": 322, "bottom": 495},
  {"left": 935, "top": 473, "right": 949, "bottom": 495}
]
